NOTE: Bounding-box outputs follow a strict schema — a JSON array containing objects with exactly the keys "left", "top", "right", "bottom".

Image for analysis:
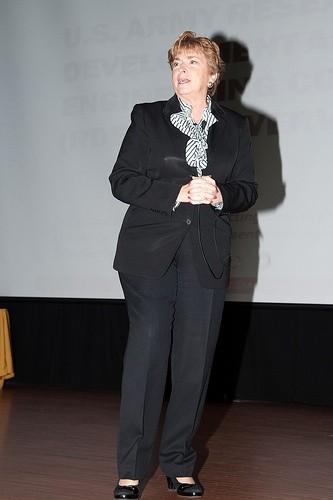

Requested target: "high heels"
[
  {"left": 112, "top": 477, "right": 143, "bottom": 498},
  {"left": 166, "top": 476, "right": 202, "bottom": 497}
]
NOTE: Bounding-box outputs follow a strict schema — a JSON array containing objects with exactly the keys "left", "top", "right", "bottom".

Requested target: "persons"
[{"left": 106, "top": 30, "right": 259, "bottom": 500}]
